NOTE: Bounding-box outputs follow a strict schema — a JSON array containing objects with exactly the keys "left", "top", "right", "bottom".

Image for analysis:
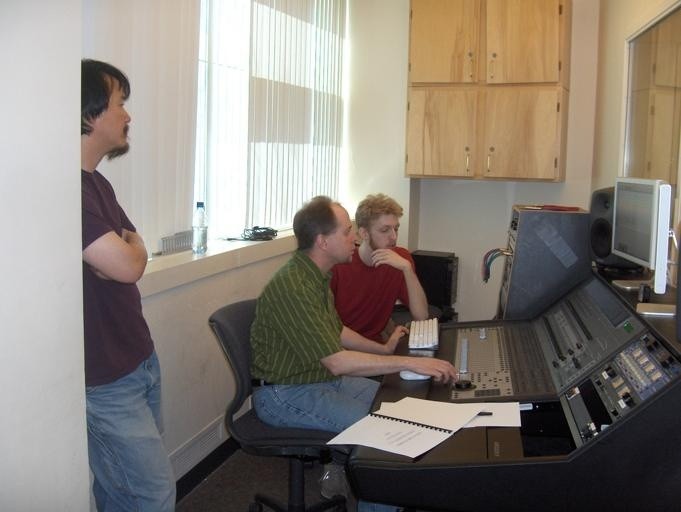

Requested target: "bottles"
[{"left": 192, "top": 200, "right": 207, "bottom": 255}]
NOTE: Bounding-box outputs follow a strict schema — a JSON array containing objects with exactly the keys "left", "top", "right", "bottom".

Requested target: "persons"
[
  {"left": 321, "top": 195, "right": 430, "bottom": 499},
  {"left": 82, "top": 59, "right": 178, "bottom": 512},
  {"left": 251, "top": 195, "right": 455, "bottom": 511}
]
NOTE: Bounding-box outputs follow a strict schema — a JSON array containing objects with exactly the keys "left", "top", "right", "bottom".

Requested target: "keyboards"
[{"left": 408, "top": 318, "right": 439, "bottom": 350}]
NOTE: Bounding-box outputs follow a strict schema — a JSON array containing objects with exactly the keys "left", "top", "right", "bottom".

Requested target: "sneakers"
[{"left": 320, "top": 464, "right": 348, "bottom": 500}]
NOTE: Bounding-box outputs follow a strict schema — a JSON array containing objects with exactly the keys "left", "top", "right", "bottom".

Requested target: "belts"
[{"left": 251, "top": 378, "right": 269, "bottom": 387}]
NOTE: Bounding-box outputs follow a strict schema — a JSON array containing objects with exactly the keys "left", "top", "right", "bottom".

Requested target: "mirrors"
[{"left": 620, "top": 2, "right": 681, "bottom": 290}]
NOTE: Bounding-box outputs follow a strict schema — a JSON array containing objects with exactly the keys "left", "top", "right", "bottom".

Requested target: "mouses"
[{"left": 399, "top": 368, "right": 431, "bottom": 380}]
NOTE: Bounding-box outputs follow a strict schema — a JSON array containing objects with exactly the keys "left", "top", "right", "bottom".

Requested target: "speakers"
[{"left": 588, "top": 186, "right": 644, "bottom": 276}]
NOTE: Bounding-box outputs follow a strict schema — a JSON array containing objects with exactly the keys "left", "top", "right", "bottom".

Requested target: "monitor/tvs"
[{"left": 610, "top": 177, "right": 671, "bottom": 294}]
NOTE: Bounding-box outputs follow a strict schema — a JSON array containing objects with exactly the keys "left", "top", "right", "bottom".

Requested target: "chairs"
[{"left": 207, "top": 299, "right": 351, "bottom": 510}]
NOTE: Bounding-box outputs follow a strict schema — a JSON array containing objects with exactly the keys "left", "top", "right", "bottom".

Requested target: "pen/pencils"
[{"left": 476, "top": 412, "right": 493, "bottom": 417}]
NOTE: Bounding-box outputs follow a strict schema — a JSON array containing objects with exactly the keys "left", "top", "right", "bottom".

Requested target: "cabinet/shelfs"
[
  {"left": 406, "top": 0, "right": 572, "bottom": 183},
  {"left": 628, "top": 9, "right": 681, "bottom": 182}
]
